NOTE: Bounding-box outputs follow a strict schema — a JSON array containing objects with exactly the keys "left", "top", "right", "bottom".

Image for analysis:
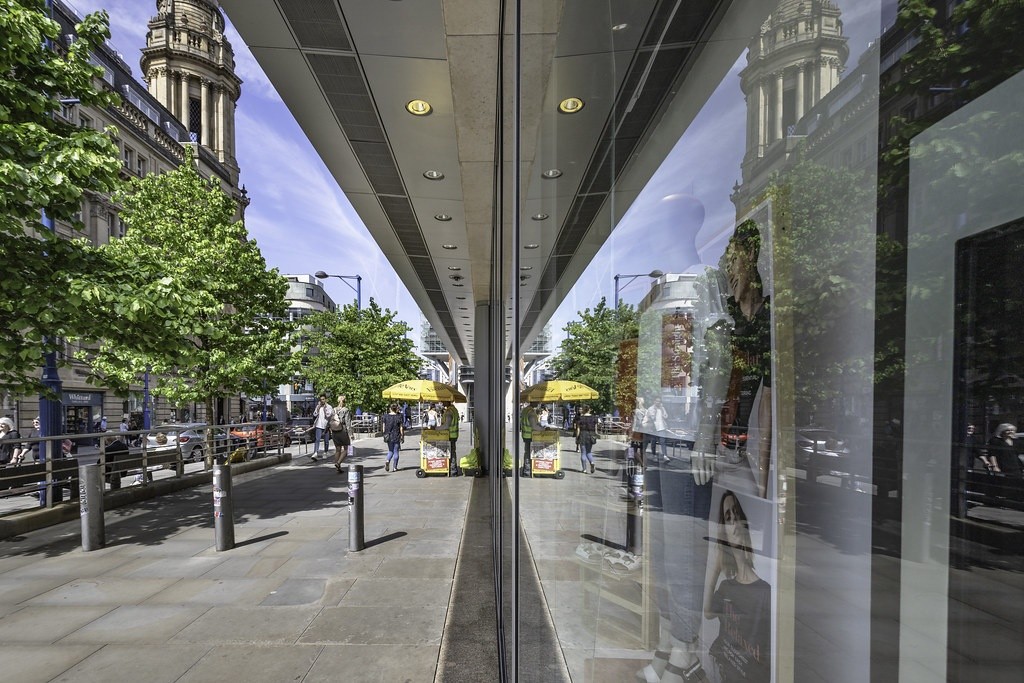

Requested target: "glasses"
[{"left": 32, "top": 421, "right": 38, "bottom": 424}]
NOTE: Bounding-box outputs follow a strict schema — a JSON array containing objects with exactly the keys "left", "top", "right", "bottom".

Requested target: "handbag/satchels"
[
  {"left": 347, "top": 439, "right": 354, "bottom": 457},
  {"left": 384, "top": 434, "right": 390, "bottom": 443},
  {"left": 590, "top": 435, "right": 596, "bottom": 444}
]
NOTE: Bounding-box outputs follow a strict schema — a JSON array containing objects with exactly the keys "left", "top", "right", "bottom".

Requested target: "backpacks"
[{"left": 329, "top": 406, "right": 346, "bottom": 431}]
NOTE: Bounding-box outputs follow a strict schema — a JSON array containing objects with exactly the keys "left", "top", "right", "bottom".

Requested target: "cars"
[
  {"left": 231, "top": 422, "right": 292, "bottom": 451},
  {"left": 715, "top": 426, "right": 750, "bottom": 449},
  {"left": 795, "top": 433, "right": 849, "bottom": 468},
  {"left": 141, "top": 422, "right": 239, "bottom": 464},
  {"left": 284, "top": 417, "right": 331, "bottom": 444}
]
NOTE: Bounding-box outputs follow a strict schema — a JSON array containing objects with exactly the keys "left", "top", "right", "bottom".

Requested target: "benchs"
[
  {"left": 105, "top": 448, "right": 184, "bottom": 490},
  {"left": 0, "top": 458, "right": 78, "bottom": 504}
]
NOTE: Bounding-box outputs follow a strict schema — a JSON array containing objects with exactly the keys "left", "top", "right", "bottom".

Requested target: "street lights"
[
  {"left": 614, "top": 268, "right": 664, "bottom": 421},
  {"left": 315, "top": 270, "right": 363, "bottom": 421}
]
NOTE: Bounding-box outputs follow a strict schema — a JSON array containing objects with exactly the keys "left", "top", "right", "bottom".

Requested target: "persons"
[
  {"left": 0, "top": 415, "right": 42, "bottom": 492},
  {"left": 461, "top": 412, "right": 465, "bottom": 423},
  {"left": 714, "top": 218, "right": 772, "bottom": 500},
  {"left": 572, "top": 406, "right": 598, "bottom": 474},
  {"left": 381, "top": 401, "right": 404, "bottom": 472},
  {"left": 404, "top": 404, "right": 414, "bottom": 429},
  {"left": 311, "top": 394, "right": 355, "bottom": 472},
  {"left": 435, "top": 401, "right": 459, "bottom": 477},
  {"left": 561, "top": 406, "right": 570, "bottom": 430},
  {"left": 423, "top": 403, "right": 437, "bottom": 430},
  {"left": 629, "top": 396, "right": 671, "bottom": 470},
  {"left": 961, "top": 421, "right": 1024, "bottom": 508},
  {"left": 97, "top": 430, "right": 170, "bottom": 486},
  {"left": 100, "top": 416, "right": 108, "bottom": 432},
  {"left": 120, "top": 417, "right": 141, "bottom": 446},
  {"left": 521, "top": 402, "right": 549, "bottom": 476},
  {"left": 218, "top": 413, "right": 247, "bottom": 432},
  {"left": 631, "top": 193, "right": 735, "bottom": 683},
  {"left": 703, "top": 489, "right": 772, "bottom": 683}
]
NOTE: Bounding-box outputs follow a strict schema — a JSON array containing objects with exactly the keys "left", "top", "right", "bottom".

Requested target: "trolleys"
[
  {"left": 532, "top": 430, "right": 566, "bottom": 479},
  {"left": 415, "top": 430, "right": 450, "bottom": 477}
]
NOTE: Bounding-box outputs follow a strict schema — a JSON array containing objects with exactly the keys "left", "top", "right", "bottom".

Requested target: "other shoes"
[
  {"left": 311, "top": 453, "right": 318, "bottom": 461},
  {"left": 664, "top": 455, "right": 670, "bottom": 464},
  {"left": 582, "top": 470, "right": 586, "bottom": 473},
  {"left": 393, "top": 468, "right": 399, "bottom": 472},
  {"left": 334, "top": 462, "right": 344, "bottom": 473},
  {"left": 322, "top": 451, "right": 327, "bottom": 460},
  {"left": 591, "top": 462, "right": 595, "bottom": 473},
  {"left": 652, "top": 455, "right": 659, "bottom": 463},
  {"left": 30, "top": 492, "right": 40, "bottom": 498},
  {"left": 384, "top": 461, "right": 389, "bottom": 471}
]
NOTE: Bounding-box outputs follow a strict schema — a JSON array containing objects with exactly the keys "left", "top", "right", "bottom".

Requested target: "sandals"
[{"left": 632, "top": 648, "right": 706, "bottom": 683}]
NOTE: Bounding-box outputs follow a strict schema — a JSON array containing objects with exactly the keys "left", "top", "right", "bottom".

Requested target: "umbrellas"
[
  {"left": 520, "top": 377, "right": 599, "bottom": 424},
  {"left": 381, "top": 379, "right": 467, "bottom": 430}
]
